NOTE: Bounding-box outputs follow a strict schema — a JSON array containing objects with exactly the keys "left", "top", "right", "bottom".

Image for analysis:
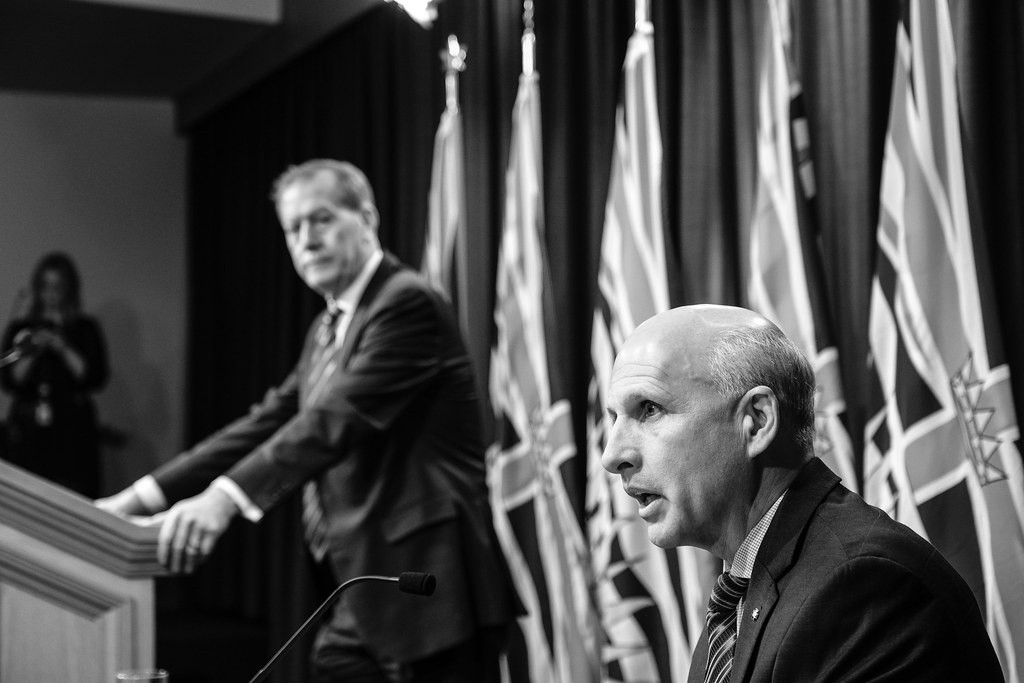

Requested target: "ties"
[
  {"left": 299, "top": 307, "right": 345, "bottom": 563},
  {"left": 702, "top": 572, "right": 749, "bottom": 683}
]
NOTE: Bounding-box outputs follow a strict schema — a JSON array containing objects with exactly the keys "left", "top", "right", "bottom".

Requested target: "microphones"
[{"left": 247, "top": 571, "right": 437, "bottom": 683}]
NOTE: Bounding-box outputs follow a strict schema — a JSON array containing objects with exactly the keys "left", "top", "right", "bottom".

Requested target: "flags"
[{"left": 419, "top": 1, "right": 1024, "bottom": 683}]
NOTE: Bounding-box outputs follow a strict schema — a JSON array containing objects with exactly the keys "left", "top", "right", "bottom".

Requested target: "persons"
[
  {"left": 0, "top": 255, "right": 109, "bottom": 497},
  {"left": 600, "top": 304, "right": 1006, "bottom": 683},
  {"left": 85, "top": 159, "right": 501, "bottom": 683}
]
